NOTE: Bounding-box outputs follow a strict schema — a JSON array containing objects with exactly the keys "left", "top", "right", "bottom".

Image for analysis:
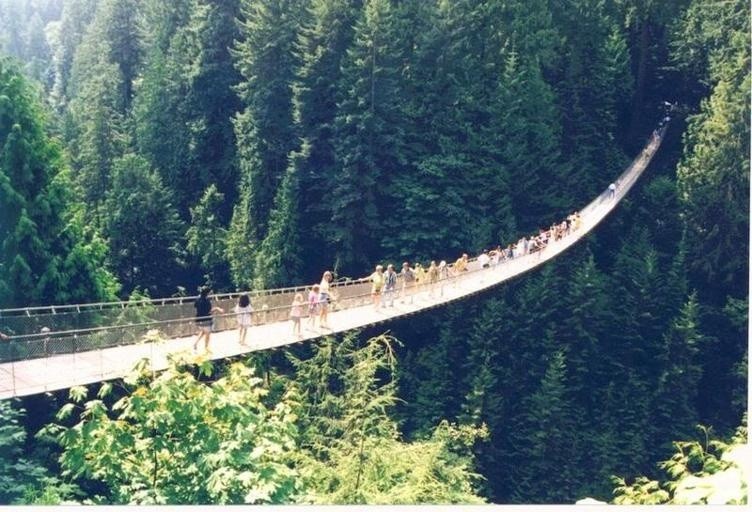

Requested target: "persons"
[
  {"left": 357, "top": 251, "right": 467, "bottom": 311},
  {"left": 638, "top": 109, "right": 672, "bottom": 166},
  {"left": 478, "top": 213, "right": 583, "bottom": 269},
  {"left": 236, "top": 292, "right": 255, "bottom": 348},
  {"left": 190, "top": 286, "right": 225, "bottom": 358},
  {"left": 307, "top": 283, "right": 320, "bottom": 333},
  {"left": 608, "top": 182, "right": 616, "bottom": 199},
  {"left": 288, "top": 293, "right": 304, "bottom": 339},
  {"left": 317, "top": 271, "right": 342, "bottom": 332}
]
